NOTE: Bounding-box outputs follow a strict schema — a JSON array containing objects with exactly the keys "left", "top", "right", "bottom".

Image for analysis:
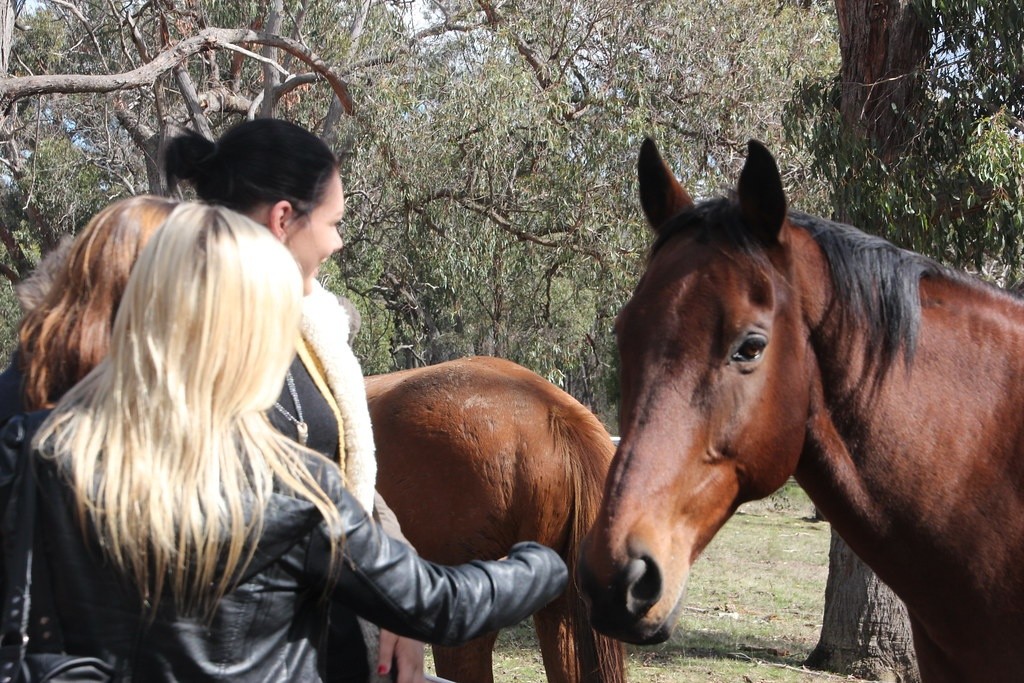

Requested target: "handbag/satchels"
[{"left": 0, "top": 630, "right": 135, "bottom": 683}]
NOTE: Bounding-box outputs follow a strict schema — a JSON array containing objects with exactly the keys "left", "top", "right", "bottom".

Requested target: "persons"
[{"left": 0, "top": 118, "right": 570, "bottom": 683}]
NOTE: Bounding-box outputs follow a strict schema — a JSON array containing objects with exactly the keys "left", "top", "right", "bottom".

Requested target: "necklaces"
[{"left": 271, "top": 368, "right": 309, "bottom": 447}]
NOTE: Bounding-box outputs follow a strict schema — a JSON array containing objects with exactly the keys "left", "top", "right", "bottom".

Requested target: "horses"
[
  {"left": 571, "top": 135, "right": 1024, "bottom": 683},
  {"left": 363, "top": 355, "right": 629, "bottom": 683}
]
